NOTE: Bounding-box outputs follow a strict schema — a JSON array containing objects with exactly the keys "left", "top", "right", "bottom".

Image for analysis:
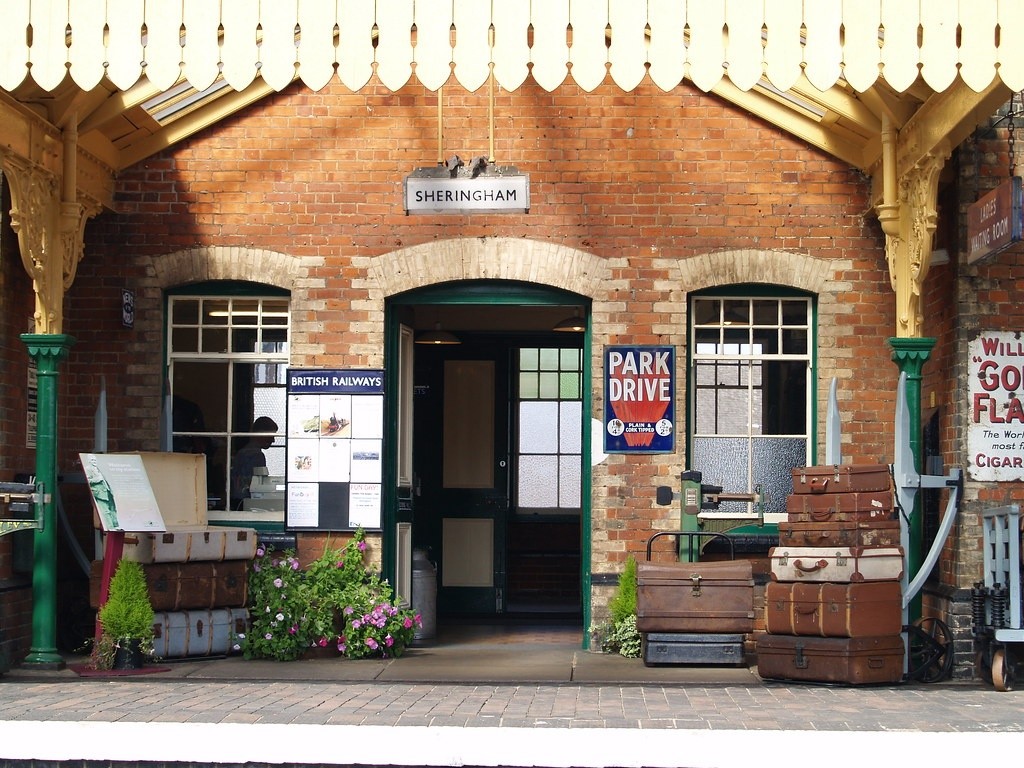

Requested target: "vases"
[{"left": 311, "top": 607, "right": 344, "bottom": 657}]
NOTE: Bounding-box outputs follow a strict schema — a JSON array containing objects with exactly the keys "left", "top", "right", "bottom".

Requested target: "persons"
[
  {"left": 233, "top": 415, "right": 279, "bottom": 511},
  {"left": 84, "top": 454, "right": 125, "bottom": 532}
]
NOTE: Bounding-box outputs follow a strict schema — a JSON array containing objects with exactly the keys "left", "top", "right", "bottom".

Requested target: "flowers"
[
  {"left": 335, "top": 575, "right": 423, "bottom": 659},
  {"left": 303, "top": 525, "right": 369, "bottom": 646},
  {"left": 230, "top": 542, "right": 306, "bottom": 662}
]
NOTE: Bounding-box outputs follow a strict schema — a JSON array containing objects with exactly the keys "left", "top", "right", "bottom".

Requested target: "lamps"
[
  {"left": 704, "top": 311, "right": 748, "bottom": 325},
  {"left": 552, "top": 307, "right": 584, "bottom": 332},
  {"left": 414, "top": 306, "right": 461, "bottom": 344}
]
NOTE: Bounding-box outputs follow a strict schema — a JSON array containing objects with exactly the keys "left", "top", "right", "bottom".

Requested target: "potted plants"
[{"left": 99, "top": 553, "right": 154, "bottom": 669}]
